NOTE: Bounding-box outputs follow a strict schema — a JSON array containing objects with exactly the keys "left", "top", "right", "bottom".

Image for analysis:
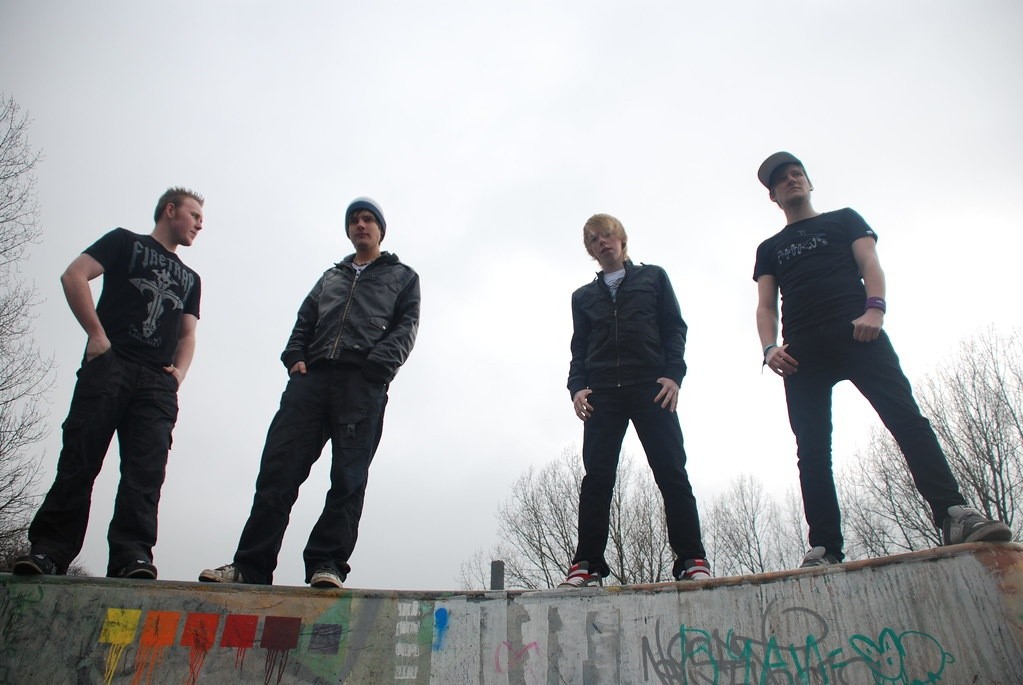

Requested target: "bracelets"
[{"left": 762, "top": 344, "right": 778, "bottom": 356}]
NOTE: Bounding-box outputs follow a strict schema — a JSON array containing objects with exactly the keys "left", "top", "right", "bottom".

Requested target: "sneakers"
[
  {"left": 195, "top": 562, "right": 273, "bottom": 587},
  {"left": 671, "top": 558, "right": 712, "bottom": 582},
  {"left": 796, "top": 545, "right": 841, "bottom": 569},
  {"left": 305, "top": 565, "right": 344, "bottom": 589},
  {"left": 105, "top": 557, "right": 157, "bottom": 580},
  {"left": 552, "top": 558, "right": 603, "bottom": 591},
  {"left": 933, "top": 504, "right": 1012, "bottom": 545},
  {"left": 12, "top": 550, "right": 68, "bottom": 580}
]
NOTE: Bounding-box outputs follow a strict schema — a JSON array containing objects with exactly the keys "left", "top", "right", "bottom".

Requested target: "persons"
[
  {"left": 13, "top": 187, "right": 207, "bottom": 579},
  {"left": 749, "top": 149, "right": 1014, "bottom": 577},
  {"left": 190, "top": 195, "right": 421, "bottom": 590},
  {"left": 553, "top": 215, "right": 712, "bottom": 589}
]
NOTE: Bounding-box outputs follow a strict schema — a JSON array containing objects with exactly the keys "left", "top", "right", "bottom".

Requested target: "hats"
[
  {"left": 344, "top": 196, "right": 387, "bottom": 240},
  {"left": 755, "top": 149, "right": 803, "bottom": 190}
]
